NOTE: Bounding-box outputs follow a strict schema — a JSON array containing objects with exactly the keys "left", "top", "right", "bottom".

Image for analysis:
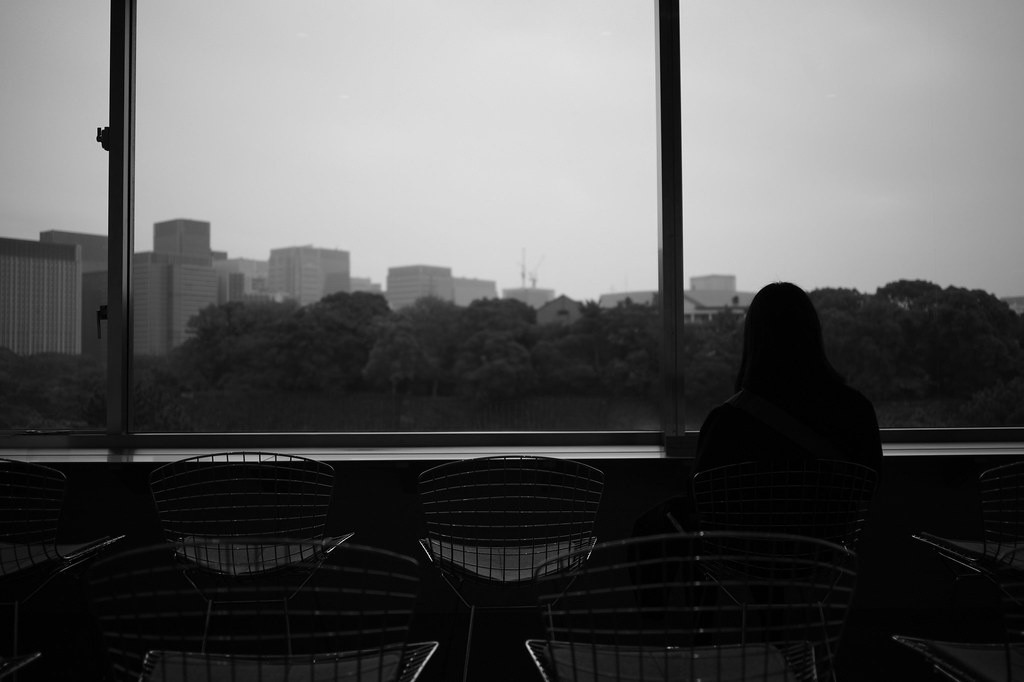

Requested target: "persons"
[{"left": 693, "top": 282, "right": 883, "bottom": 474}]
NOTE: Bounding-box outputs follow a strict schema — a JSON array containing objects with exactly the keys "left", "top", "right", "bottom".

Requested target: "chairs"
[{"left": 0, "top": 450, "right": 1024, "bottom": 682}]
